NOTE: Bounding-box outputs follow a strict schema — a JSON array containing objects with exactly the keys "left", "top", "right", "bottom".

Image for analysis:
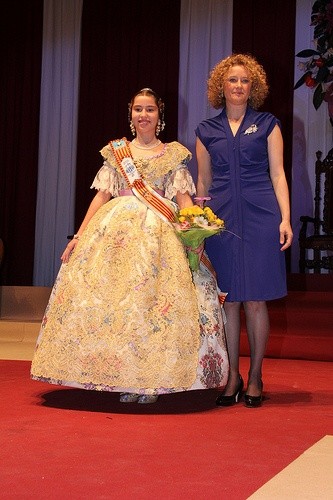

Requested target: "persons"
[
  {"left": 195, "top": 54, "right": 293, "bottom": 408},
  {"left": 30, "top": 87, "right": 230, "bottom": 403}
]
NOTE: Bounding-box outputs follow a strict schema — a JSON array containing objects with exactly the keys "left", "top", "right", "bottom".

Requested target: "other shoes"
[
  {"left": 120, "top": 394, "right": 138, "bottom": 402},
  {"left": 138, "top": 396, "right": 158, "bottom": 403}
]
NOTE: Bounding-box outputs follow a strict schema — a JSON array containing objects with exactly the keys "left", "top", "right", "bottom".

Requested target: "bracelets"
[{"left": 72, "top": 234, "right": 80, "bottom": 240}]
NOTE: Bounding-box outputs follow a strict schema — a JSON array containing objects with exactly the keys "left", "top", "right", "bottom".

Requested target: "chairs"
[{"left": 298, "top": 147, "right": 333, "bottom": 273}]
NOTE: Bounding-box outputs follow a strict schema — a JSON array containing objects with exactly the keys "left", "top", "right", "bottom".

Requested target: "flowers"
[{"left": 172, "top": 196, "right": 225, "bottom": 271}]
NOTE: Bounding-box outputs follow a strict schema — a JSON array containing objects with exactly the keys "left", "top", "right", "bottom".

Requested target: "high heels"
[
  {"left": 244, "top": 380, "right": 263, "bottom": 405},
  {"left": 216, "top": 379, "right": 243, "bottom": 405}
]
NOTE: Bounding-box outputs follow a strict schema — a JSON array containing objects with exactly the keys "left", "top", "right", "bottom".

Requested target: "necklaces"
[{"left": 131, "top": 138, "right": 161, "bottom": 150}]
